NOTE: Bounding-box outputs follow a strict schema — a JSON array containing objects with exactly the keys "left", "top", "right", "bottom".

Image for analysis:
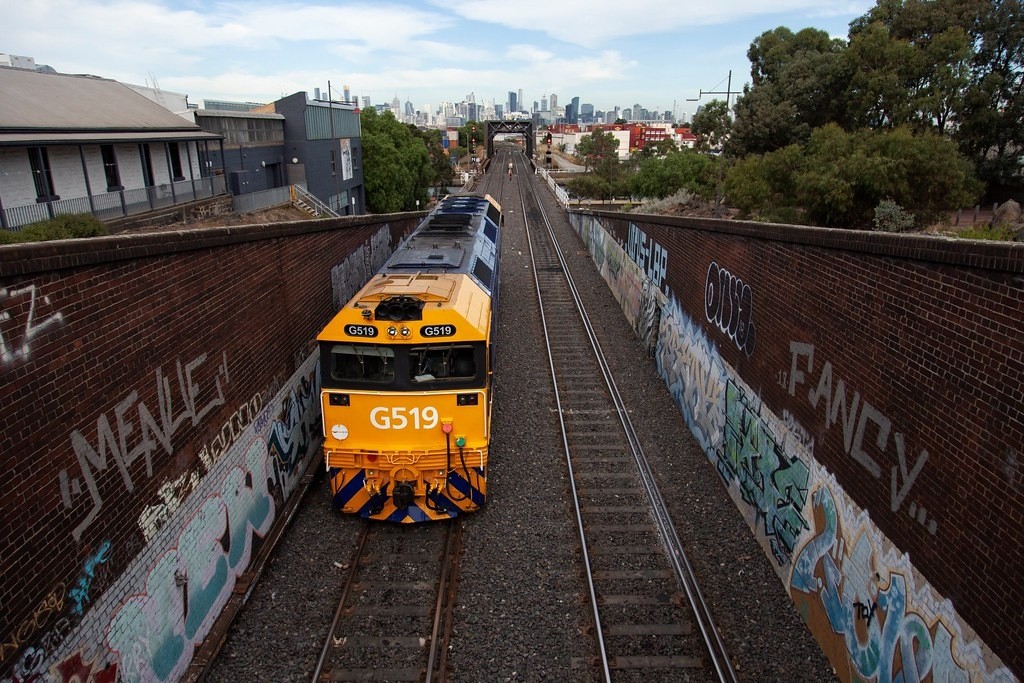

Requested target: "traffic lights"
[
  {"left": 546, "top": 133, "right": 552, "bottom": 146},
  {"left": 471, "top": 125, "right": 476, "bottom": 131},
  {"left": 471, "top": 133, "right": 477, "bottom": 142},
  {"left": 546, "top": 124, "right": 552, "bottom": 130}
]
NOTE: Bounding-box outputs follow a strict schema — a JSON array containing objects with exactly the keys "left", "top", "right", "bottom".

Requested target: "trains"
[{"left": 315, "top": 192, "right": 505, "bottom": 526}]
